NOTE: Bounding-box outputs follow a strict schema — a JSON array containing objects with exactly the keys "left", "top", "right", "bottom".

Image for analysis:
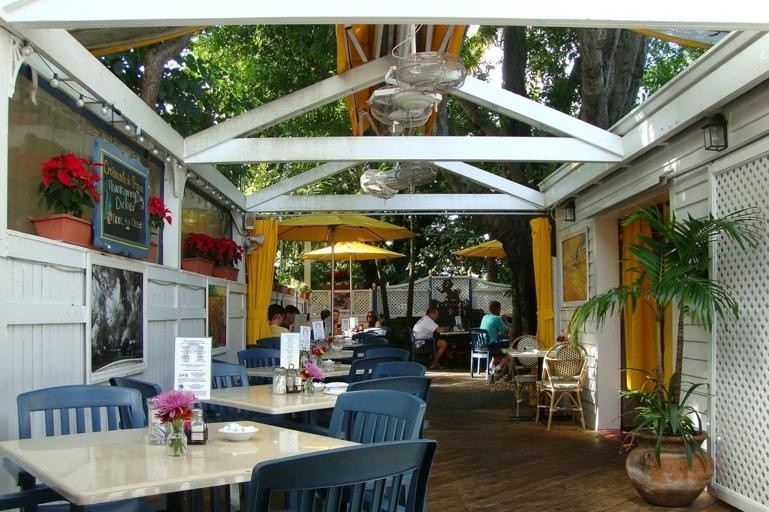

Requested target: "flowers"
[
  {"left": 183, "top": 232, "right": 243, "bottom": 265},
  {"left": 151, "top": 388, "right": 196, "bottom": 453},
  {"left": 37, "top": 153, "right": 104, "bottom": 216},
  {"left": 149, "top": 195, "right": 173, "bottom": 231}
]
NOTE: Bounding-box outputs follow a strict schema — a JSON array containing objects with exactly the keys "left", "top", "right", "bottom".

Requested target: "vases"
[
  {"left": 145, "top": 243, "right": 158, "bottom": 262},
  {"left": 29, "top": 214, "right": 92, "bottom": 247},
  {"left": 167, "top": 419, "right": 188, "bottom": 459},
  {"left": 181, "top": 258, "right": 240, "bottom": 281}
]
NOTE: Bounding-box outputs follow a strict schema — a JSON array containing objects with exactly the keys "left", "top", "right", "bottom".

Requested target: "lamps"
[
  {"left": 700, "top": 113, "right": 729, "bottom": 152},
  {"left": 245, "top": 234, "right": 265, "bottom": 255},
  {"left": 563, "top": 200, "right": 576, "bottom": 221}
]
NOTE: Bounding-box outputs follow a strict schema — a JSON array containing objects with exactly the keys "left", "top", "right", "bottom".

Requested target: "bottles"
[
  {"left": 190, "top": 409, "right": 207, "bottom": 444},
  {"left": 145, "top": 397, "right": 166, "bottom": 444},
  {"left": 271, "top": 364, "right": 302, "bottom": 395}
]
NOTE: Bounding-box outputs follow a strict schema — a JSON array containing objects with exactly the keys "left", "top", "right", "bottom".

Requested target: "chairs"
[
  {"left": 296, "top": 360, "right": 431, "bottom": 443},
  {"left": 246, "top": 439, "right": 439, "bottom": 511},
  {"left": 210, "top": 362, "right": 251, "bottom": 388},
  {"left": 509, "top": 334, "right": 548, "bottom": 416},
  {"left": 234, "top": 329, "right": 431, "bottom": 382},
  {"left": 1, "top": 385, "right": 147, "bottom": 512},
  {"left": 467, "top": 327, "right": 503, "bottom": 380},
  {"left": 109, "top": 376, "right": 162, "bottom": 429},
  {"left": 536, "top": 341, "right": 588, "bottom": 432}
]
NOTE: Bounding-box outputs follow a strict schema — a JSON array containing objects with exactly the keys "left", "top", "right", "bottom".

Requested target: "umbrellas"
[
  {"left": 454, "top": 239, "right": 508, "bottom": 258},
  {"left": 277, "top": 211, "right": 421, "bottom": 338},
  {"left": 301, "top": 241, "right": 406, "bottom": 318}
]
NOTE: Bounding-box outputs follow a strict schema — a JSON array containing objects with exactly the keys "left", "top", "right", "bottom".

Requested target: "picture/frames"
[
  {"left": 205, "top": 277, "right": 228, "bottom": 356},
  {"left": 87, "top": 254, "right": 145, "bottom": 384}
]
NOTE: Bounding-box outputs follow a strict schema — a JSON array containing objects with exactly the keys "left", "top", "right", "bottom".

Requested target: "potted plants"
[
  {"left": 565, "top": 200, "right": 764, "bottom": 508},
  {"left": 272, "top": 270, "right": 312, "bottom": 299}
]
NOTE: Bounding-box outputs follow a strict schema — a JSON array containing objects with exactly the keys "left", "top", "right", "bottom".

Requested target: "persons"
[
  {"left": 268, "top": 304, "right": 344, "bottom": 338},
  {"left": 362, "top": 311, "right": 381, "bottom": 329},
  {"left": 412, "top": 307, "right": 449, "bottom": 369},
  {"left": 476, "top": 301, "right": 509, "bottom": 376}
]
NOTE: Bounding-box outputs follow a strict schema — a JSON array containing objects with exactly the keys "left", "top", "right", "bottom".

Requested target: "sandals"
[{"left": 430, "top": 363, "right": 445, "bottom": 369}]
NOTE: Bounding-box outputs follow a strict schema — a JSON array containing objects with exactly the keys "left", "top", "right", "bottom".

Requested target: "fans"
[{"left": 361, "top": 23, "right": 468, "bottom": 200}]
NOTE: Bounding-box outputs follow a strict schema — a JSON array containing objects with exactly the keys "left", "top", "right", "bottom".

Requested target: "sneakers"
[{"left": 485, "top": 368, "right": 502, "bottom": 375}]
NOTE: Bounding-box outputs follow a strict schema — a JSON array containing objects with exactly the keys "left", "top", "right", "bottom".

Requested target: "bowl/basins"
[
  {"left": 217, "top": 441, "right": 257, "bottom": 455},
  {"left": 303, "top": 381, "right": 351, "bottom": 395},
  {"left": 218, "top": 426, "right": 261, "bottom": 440}
]
